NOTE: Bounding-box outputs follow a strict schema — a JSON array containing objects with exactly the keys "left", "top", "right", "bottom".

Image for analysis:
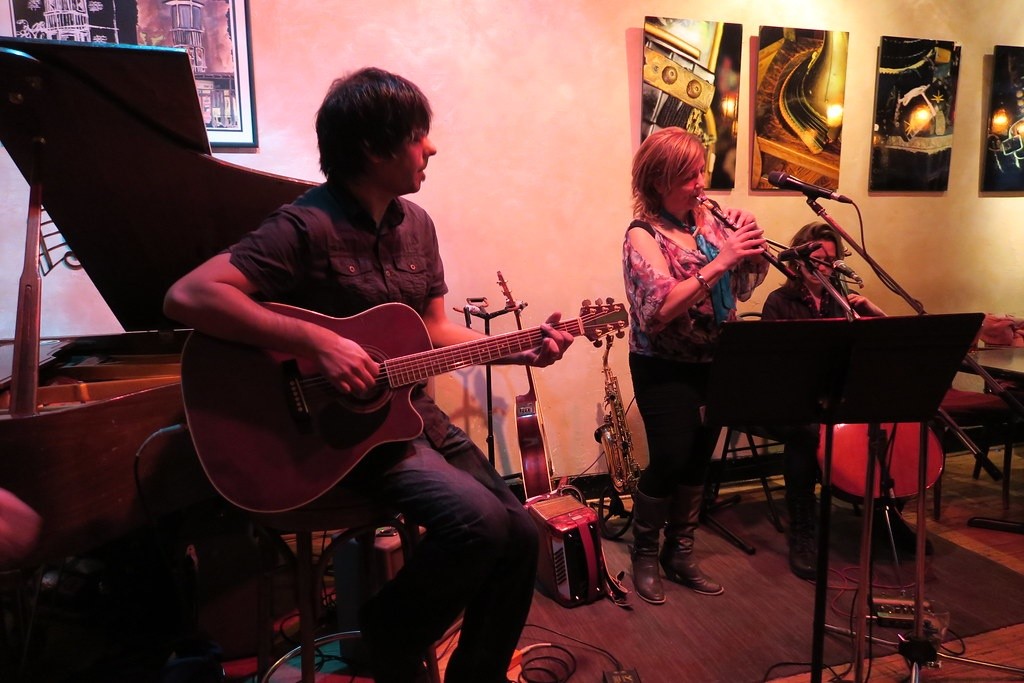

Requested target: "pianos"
[{"left": 0, "top": 31, "right": 327, "bottom": 683}]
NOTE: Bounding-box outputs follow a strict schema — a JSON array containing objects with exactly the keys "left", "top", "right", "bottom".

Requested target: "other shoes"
[{"left": 360, "top": 620, "right": 432, "bottom": 683}]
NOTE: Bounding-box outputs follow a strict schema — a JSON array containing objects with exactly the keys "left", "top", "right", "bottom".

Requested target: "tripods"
[
  {"left": 589, "top": 477, "right": 633, "bottom": 522},
  {"left": 803, "top": 255, "right": 1024, "bottom": 683}
]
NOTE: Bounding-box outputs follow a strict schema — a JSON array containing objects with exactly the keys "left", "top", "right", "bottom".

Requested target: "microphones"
[
  {"left": 832, "top": 260, "right": 860, "bottom": 282},
  {"left": 767, "top": 172, "right": 852, "bottom": 204},
  {"left": 778, "top": 241, "right": 823, "bottom": 262}
]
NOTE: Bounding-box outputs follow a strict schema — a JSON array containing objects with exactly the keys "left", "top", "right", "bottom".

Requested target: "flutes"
[{"left": 695, "top": 194, "right": 797, "bottom": 282}]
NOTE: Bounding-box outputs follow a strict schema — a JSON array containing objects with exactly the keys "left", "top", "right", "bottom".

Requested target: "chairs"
[{"left": 713, "top": 420, "right": 789, "bottom": 531}]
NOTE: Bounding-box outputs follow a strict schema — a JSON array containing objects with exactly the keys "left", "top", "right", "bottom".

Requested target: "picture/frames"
[{"left": 0, "top": 0, "right": 260, "bottom": 149}]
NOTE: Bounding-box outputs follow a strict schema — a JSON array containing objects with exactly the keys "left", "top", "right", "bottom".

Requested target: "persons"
[
  {"left": 763, "top": 221, "right": 895, "bottom": 580},
  {"left": 161, "top": 65, "right": 573, "bottom": 683},
  {"left": 624, "top": 126, "right": 772, "bottom": 603}
]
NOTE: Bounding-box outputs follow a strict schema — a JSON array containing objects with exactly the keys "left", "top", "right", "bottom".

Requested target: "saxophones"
[{"left": 593, "top": 337, "right": 643, "bottom": 497}]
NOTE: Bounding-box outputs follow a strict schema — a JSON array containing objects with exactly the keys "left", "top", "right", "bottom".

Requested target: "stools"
[
  {"left": 253, "top": 503, "right": 442, "bottom": 683},
  {"left": 935, "top": 388, "right": 1016, "bottom": 520}
]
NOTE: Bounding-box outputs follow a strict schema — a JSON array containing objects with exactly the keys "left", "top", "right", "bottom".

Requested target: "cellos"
[{"left": 817, "top": 241, "right": 946, "bottom": 594}]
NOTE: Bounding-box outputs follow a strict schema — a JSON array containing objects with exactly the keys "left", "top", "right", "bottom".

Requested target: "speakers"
[{"left": 529, "top": 494, "right": 608, "bottom": 607}]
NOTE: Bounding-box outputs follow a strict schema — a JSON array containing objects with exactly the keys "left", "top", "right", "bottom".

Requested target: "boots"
[
  {"left": 660, "top": 484, "right": 724, "bottom": 595},
  {"left": 872, "top": 496, "right": 934, "bottom": 556},
  {"left": 784, "top": 495, "right": 818, "bottom": 580},
  {"left": 627, "top": 480, "right": 670, "bottom": 603}
]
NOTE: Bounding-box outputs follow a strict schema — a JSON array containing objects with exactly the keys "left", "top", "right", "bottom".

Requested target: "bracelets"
[{"left": 695, "top": 271, "right": 713, "bottom": 291}]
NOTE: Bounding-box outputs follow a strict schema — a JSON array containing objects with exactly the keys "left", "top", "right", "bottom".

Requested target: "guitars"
[
  {"left": 177, "top": 296, "right": 631, "bottom": 516},
  {"left": 495, "top": 269, "right": 555, "bottom": 503}
]
